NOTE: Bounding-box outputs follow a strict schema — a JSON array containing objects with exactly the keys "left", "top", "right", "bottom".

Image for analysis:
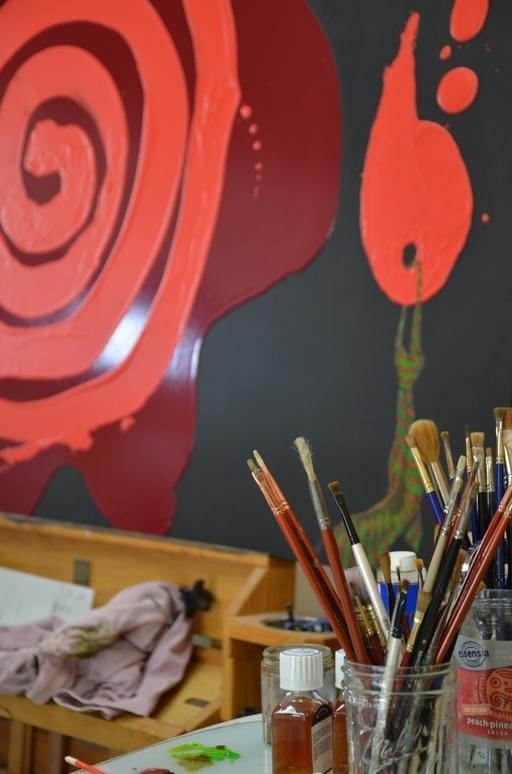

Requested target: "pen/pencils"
[
  {"left": 64, "top": 755, "right": 108, "bottom": 774},
  {"left": 287, "top": 603, "right": 294, "bottom": 621}
]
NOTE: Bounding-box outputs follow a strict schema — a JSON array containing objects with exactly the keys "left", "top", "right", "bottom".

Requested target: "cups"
[
  {"left": 454, "top": 587, "right": 512, "bottom": 774},
  {"left": 340, "top": 652, "right": 459, "bottom": 774}
]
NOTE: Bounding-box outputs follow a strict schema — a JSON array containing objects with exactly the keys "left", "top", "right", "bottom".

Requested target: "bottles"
[
  {"left": 260, "top": 640, "right": 350, "bottom": 774},
  {"left": 375, "top": 551, "right": 428, "bottom": 634}
]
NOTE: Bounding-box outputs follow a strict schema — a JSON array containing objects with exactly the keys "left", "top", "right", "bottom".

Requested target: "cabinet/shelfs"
[{"left": 0, "top": 514, "right": 293, "bottom": 774}]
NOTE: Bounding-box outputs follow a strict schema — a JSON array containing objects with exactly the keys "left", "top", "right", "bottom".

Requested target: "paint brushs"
[{"left": 245, "top": 406, "right": 512, "bottom": 774}]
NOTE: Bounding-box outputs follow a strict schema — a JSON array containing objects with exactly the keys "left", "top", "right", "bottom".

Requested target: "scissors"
[{"left": 285, "top": 619, "right": 332, "bottom": 633}]
